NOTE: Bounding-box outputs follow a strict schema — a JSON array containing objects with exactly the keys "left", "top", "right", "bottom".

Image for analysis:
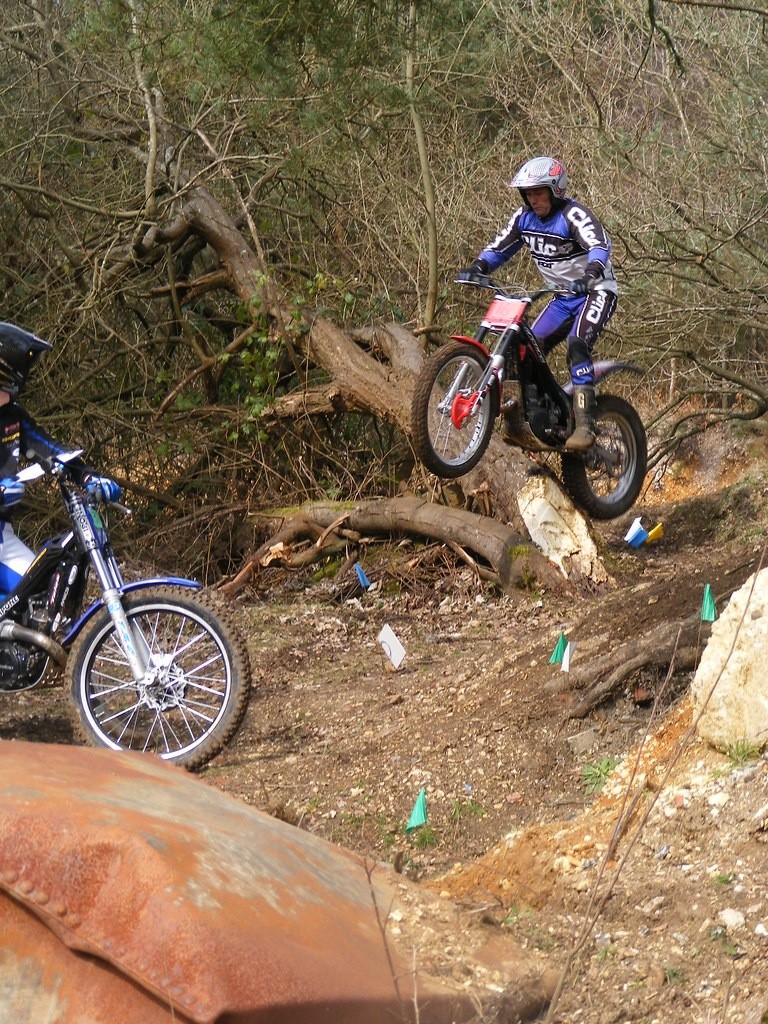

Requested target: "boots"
[{"left": 565, "top": 384, "right": 596, "bottom": 450}]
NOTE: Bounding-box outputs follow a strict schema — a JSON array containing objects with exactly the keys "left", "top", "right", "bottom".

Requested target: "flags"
[
  {"left": 548, "top": 632, "right": 568, "bottom": 664},
  {"left": 702, "top": 584, "right": 716, "bottom": 621},
  {"left": 406, "top": 787, "right": 427, "bottom": 835},
  {"left": 560, "top": 642, "right": 577, "bottom": 672},
  {"left": 624, "top": 517, "right": 665, "bottom": 548},
  {"left": 354, "top": 562, "right": 370, "bottom": 587},
  {"left": 378, "top": 623, "right": 407, "bottom": 670}
]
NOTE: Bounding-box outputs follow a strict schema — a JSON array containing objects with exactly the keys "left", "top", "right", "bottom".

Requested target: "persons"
[
  {"left": 458, "top": 157, "right": 618, "bottom": 450},
  {"left": 0, "top": 322, "right": 120, "bottom": 603}
]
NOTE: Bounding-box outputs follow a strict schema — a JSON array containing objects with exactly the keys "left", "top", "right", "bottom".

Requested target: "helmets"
[
  {"left": 1, "top": 322, "right": 53, "bottom": 395},
  {"left": 510, "top": 157, "right": 568, "bottom": 208}
]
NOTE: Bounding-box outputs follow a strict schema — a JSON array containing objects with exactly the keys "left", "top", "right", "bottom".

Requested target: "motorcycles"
[
  {"left": 410, "top": 267, "right": 648, "bottom": 523},
  {"left": 0, "top": 447, "right": 254, "bottom": 774}
]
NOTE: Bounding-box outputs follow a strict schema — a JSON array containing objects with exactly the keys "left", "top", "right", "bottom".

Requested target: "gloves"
[
  {"left": 84, "top": 474, "right": 122, "bottom": 504},
  {"left": 0, "top": 476, "right": 25, "bottom": 510},
  {"left": 571, "top": 263, "right": 605, "bottom": 295},
  {"left": 456, "top": 260, "right": 489, "bottom": 281}
]
